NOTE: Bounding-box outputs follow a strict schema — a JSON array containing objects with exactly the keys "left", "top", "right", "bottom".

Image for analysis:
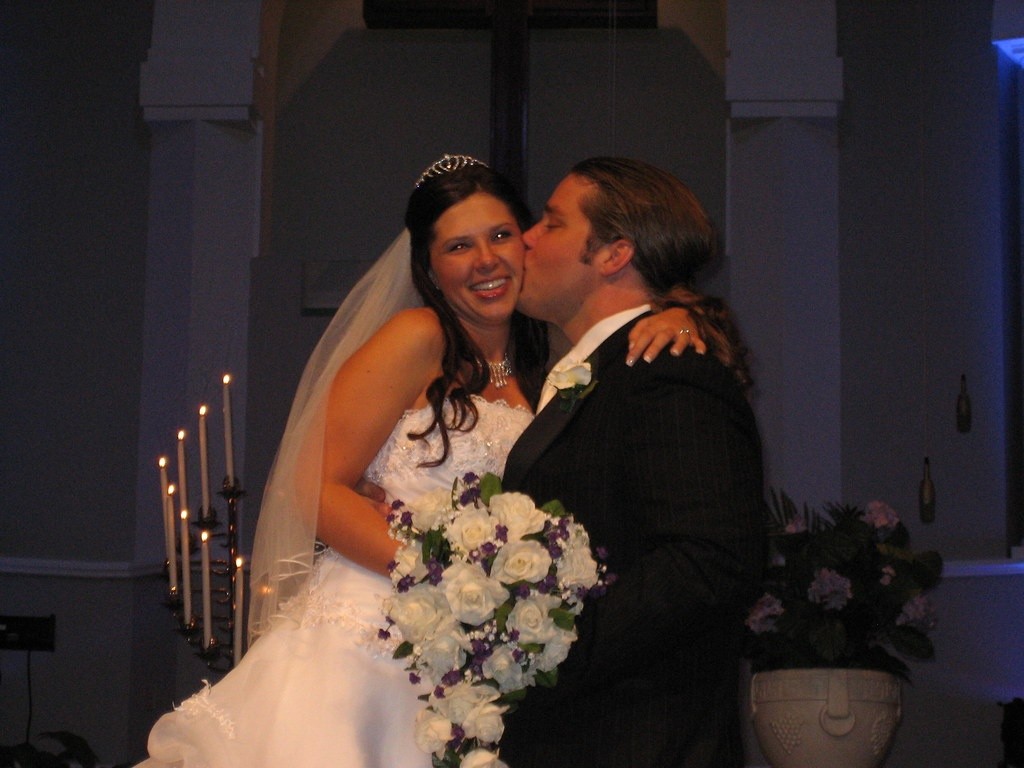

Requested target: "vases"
[{"left": 746, "top": 668, "right": 904, "bottom": 768}]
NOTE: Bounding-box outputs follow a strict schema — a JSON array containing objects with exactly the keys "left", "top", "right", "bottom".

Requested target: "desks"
[{"left": 917, "top": 557, "right": 1024, "bottom": 768}]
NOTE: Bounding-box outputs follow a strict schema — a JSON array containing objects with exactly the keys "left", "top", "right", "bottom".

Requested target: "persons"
[
  {"left": 497, "top": 160, "right": 768, "bottom": 767},
  {"left": 291, "top": 154, "right": 707, "bottom": 767}
]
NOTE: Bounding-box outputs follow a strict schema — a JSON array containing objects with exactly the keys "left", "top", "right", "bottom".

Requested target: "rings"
[{"left": 676, "top": 328, "right": 692, "bottom": 339}]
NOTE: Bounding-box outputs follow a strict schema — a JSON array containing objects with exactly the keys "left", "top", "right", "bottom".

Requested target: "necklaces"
[{"left": 474, "top": 352, "right": 513, "bottom": 388}]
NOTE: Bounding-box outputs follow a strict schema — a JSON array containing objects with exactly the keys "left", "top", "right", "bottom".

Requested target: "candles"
[
  {"left": 233, "top": 555, "right": 245, "bottom": 667},
  {"left": 200, "top": 529, "right": 212, "bottom": 649},
  {"left": 158, "top": 455, "right": 170, "bottom": 560},
  {"left": 222, "top": 372, "right": 235, "bottom": 487},
  {"left": 177, "top": 428, "right": 188, "bottom": 512},
  {"left": 167, "top": 483, "right": 177, "bottom": 591},
  {"left": 180, "top": 509, "right": 192, "bottom": 624},
  {"left": 198, "top": 404, "right": 210, "bottom": 519}
]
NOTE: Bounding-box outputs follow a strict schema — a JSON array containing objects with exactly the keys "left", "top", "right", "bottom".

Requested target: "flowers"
[
  {"left": 728, "top": 489, "right": 943, "bottom": 686},
  {"left": 366, "top": 472, "right": 616, "bottom": 768},
  {"left": 547, "top": 356, "right": 598, "bottom": 424}
]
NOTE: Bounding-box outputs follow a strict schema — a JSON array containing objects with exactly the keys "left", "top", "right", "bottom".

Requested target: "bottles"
[
  {"left": 956, "top": 373, "right": 971, "bottom": 432},
  {"left": 920, "top": 456, "right": 936, "bottom": 523}
]
